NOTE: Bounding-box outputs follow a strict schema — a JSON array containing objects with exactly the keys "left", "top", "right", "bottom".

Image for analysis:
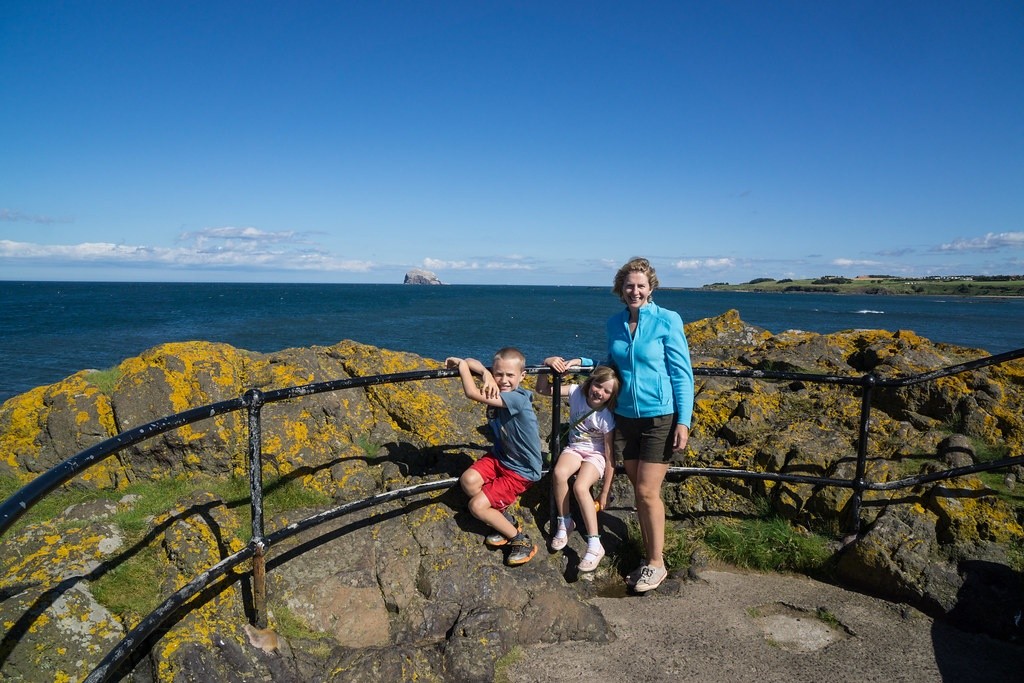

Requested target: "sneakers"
[
  {"left": 485, "top": 513, "right": 525, "bottom": 546},
  {"left": 626, "top": 559, "right": 648, "bottom": 590},
  {"left": 551, "top": 519, "right": 576, "bottom": 551},
  {"left": 577, "top": 545, "right": 605, "bottom": 571},
  {"left": 634, "top": 564, "right": 667, "bottom": 592},
  {"left": 507, "top": 535, "right": 537, "bottom": 566}
]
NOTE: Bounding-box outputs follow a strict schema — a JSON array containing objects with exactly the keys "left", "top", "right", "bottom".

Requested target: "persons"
[
  {"left": 536, "top": 357, "right": 620, "bottom": 572},
  {"left": 563, "top": 258, "right": 694, "bottom": 593},
  {"left": 445, "top": 347, "right": 542, "bottom": 566}
]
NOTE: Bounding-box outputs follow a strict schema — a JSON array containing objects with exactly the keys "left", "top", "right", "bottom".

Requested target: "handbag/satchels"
[{"left": 547, "top": 421, "right": 571, "bottom": 453}]
